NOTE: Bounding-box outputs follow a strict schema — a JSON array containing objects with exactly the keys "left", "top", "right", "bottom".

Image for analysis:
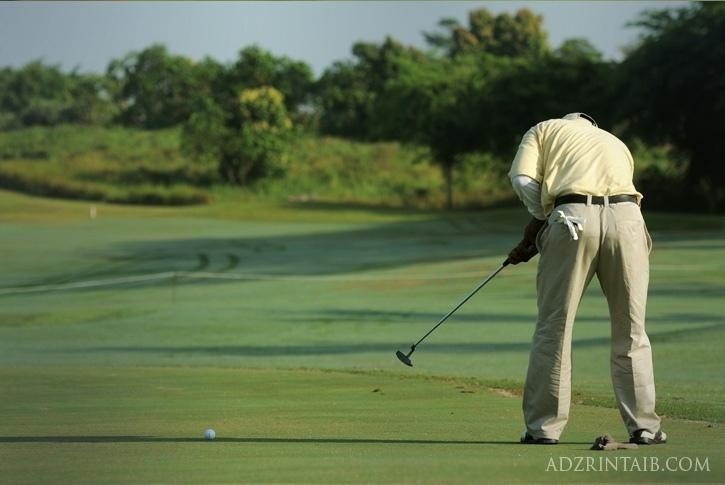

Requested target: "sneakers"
[
  {"left": 629, "top": 428, "right": 667, "bottom": 445},
  {"left": 520, "top": 431, "right": 559, "bottom": 445}
]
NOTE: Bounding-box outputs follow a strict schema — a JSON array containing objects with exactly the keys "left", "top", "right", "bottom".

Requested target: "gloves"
[{"left": 548, "top": 211, "right": 586, "bottom": 240}]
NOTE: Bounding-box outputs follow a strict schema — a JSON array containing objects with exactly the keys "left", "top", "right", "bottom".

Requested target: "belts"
[{"left": 554, "top": 193, "right": 638, "bottom": 209}]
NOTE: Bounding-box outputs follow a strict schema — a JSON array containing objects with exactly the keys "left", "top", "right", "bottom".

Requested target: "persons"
[{"left": 507, "top": 112, "right": 668, "bottom": 445}]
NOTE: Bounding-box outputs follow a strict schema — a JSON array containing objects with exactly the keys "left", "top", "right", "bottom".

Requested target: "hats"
[{"left": 562, "top": 112, "right": 598, "bottom": 128}]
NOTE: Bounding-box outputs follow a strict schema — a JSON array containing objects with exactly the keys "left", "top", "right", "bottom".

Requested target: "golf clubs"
[{"left": 396, "top": 257, "right": 511, "bottom": 366}]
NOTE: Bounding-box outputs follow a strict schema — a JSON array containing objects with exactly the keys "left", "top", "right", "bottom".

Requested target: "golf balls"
[{"left": 204, "top": 430, "right": 216, "bottom": 440}]
